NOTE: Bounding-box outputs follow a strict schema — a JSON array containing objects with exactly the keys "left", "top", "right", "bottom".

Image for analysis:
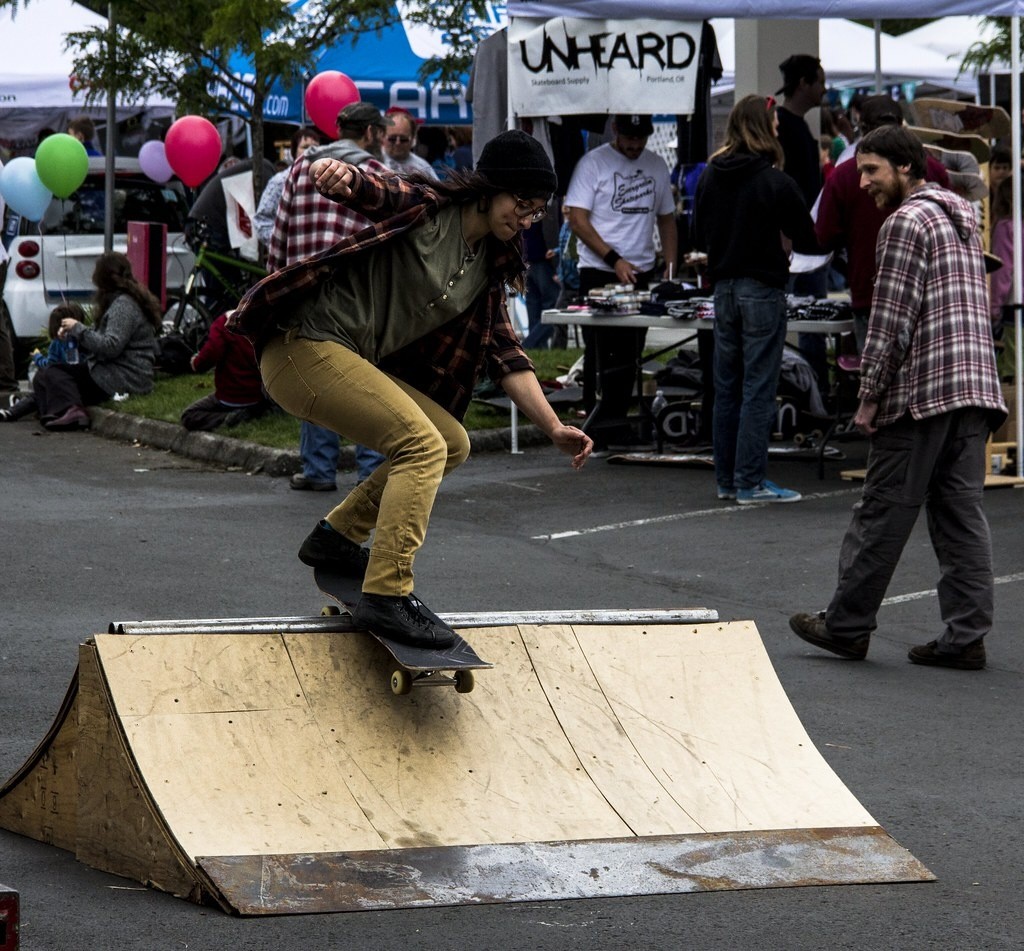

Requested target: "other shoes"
[
  {"left": 224, "top": 408, "right": 245, "bottom": 428},
  {"left": 40, "top": 414, "right": 58, "bottom": 428},
  {"left": 9, "top": 394, "right": 20, "bottom": 407},
  {"left": 0, "top": 409, "right": 16, "bottom": 422}
]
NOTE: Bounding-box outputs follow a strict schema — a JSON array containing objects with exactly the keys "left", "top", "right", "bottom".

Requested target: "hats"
[
  {"left": 338, "top": 102, "right": 395, "bottom": 129},
  {"left": 471, "top": 128, "right": 557, "bottom": 192},
  {"left": 614, "top": 113, "right": 654, "bottom": 138},
  {"left": 855, "top": 95, "right": 903, "bottom": 131},
  {"left": 773, "top": 54, "right": 821, "bottom": 95}
]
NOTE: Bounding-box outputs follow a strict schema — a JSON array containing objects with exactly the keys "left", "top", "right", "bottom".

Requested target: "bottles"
[
  {"left": 28, "top": 362, "right": 39, "bottom": 382},
  {"left": 509, "top": 290, "right": 522, "bottom": 344},
  {"left": 67, "top": 330, "right": 79, "bottom": 364},
  {"left": 284, "top": 144, "right": 293, "bottom": 161}
]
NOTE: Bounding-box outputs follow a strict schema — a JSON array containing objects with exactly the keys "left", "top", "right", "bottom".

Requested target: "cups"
[{"left": 18, "top": 381, "right": 28, "bottom": 391}]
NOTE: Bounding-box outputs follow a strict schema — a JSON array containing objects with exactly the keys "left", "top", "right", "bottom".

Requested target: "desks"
[{"left": 540, "top": 305, "right": 857, "bottom": 456}]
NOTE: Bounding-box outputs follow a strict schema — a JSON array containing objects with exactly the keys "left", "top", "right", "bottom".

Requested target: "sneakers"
[
  {"left": 717, "top": 485, "right": 735, "bottom": 498},
  {"left": 789, "top": 614, "right": 866, "bottom": 659},
  {"left": 737, "top": 479, "right": 802, "bottom": 503},
  {"left": 290, "top": 473, "right": 337, "bottom": 492},
  {"left": 299, "top": 519, "right": 372, "bottom": 579},
  {"left": 351, "top": 592, "right": 454, "bottom": 650},
  {"left": 908, "top": 640, "right": 986, "bottom": 669}
]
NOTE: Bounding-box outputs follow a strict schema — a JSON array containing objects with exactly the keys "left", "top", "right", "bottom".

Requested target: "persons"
[
  {"left": 774, "top": 55, "right": 903, "bottom": 379},
  {"left": 225, "top": 130, "right": 593, "bottom": 649},
  {"left": 789, "top": 126, "right": 1008, "bottom": 670},
  {"left": 689, "top": 95, "right": 817, "bottom": 504},
  {"left": 185, "top": 130, "right": 324, "bottom": 309},
  {"left": 519, "top": 114, "right": 707, "bottom": 459},
  {"left": 813, "top": 96, "right": 950, "bottom": 353},
  {"left": 37, "top": 117, "right": 101, "bottom": 157},
  {"left": 382, "top": 113, "right": 472, "bottom": 184},
  {"left": 180, "top": 309, "right": 272, "bottom": 432},
  {"left": 989, "top": 147, "right": 1015, "bottom": 382},
  {"left": 0, "top": 252, "right": 164, "bottom": 430},
  {"left": 266, "top": 102, "right": 397, "bottom": 491}
]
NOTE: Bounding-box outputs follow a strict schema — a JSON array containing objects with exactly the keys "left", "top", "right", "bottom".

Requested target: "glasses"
[
  {"left": 383, "top": 135, "right": 413, "bottom": 142},
  {"left": 766, "top": 96, "right": 776, "bottom": 112},
  {"left": 512, "top": 193, "right": 547, "bottom": 223}
]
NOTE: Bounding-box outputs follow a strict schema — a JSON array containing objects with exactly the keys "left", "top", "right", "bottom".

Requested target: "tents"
[
  {"left": 0, "top": 1, "right": 185, "bottom": 124},
  {"left": 507, "top": 0, "right": 1024, "bottom": 487},
  {"left": 195, "top": 0, "right": 508, "bottom": 127}
]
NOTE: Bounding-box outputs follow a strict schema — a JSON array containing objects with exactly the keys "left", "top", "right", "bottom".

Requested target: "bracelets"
[{"left": 603, "top": 249, "right": 623, "bottom": 269}]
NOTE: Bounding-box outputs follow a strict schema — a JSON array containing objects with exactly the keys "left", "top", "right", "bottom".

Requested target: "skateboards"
[
  {"left": 607, "top": 429, "right": 847, "bottom": 467},
  {"left": 312, "top": 544, "right": 495, "bottom": 696},
  {"left": 657, "top": 399, "right": 805, "bottom": 445}
]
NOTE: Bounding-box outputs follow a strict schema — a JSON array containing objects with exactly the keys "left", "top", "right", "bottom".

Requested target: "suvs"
[{"left": 0, "top": 157, "right": 269, "bottom": 380}]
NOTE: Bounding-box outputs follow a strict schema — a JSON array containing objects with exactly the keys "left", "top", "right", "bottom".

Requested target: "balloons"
[
  {"left": 304, "top": 70, "right": 361, "bottom": 139},
  {"left": 139, "top": 114, "right": 222, "bottom": 187},
  {"left": 0, "top": 133, "right": 88, "bottom": 222}
]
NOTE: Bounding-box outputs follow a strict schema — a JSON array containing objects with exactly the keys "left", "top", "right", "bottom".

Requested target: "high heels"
[{"left": 46, "top": 404, "right": 90, "bottom": 432}]
